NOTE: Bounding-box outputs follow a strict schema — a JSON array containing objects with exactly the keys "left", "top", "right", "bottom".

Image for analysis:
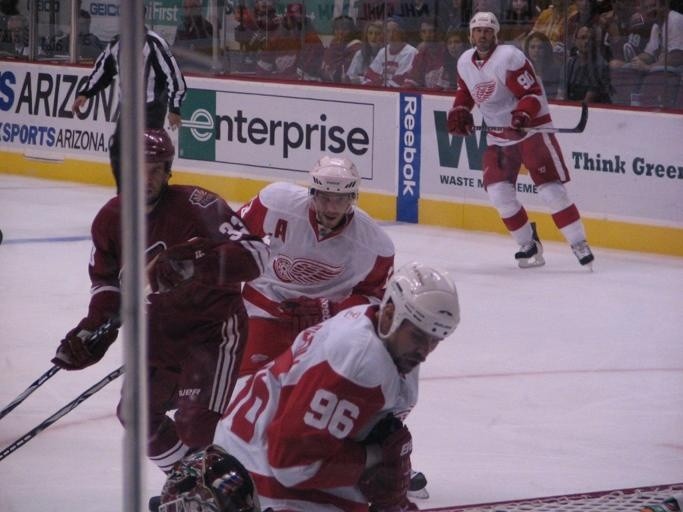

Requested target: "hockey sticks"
[{"left": 469, "top": 102, "right": 588, "bottom": 133}]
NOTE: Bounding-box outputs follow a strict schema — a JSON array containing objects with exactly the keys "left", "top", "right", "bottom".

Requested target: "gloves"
[
  {"left": 511, "top": 110, "right": 530, "bottom": 129},
  {"left": 281, "top": 296, "right": 339, "bottom": 331},
  {"left": 156, "top": 238, "right": 218, "bottom": 294},
  {"left": 360, "top": 412, "right": 412, "bottom": 501},
  {"left": 52, "top": 315, "right": 118, "bottom": 370},
  {"left": 448, "top": 108, "right": 473, "bottom": 136}
]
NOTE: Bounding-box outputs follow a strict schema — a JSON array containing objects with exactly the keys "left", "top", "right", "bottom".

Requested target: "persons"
[
  {"left": 70, "top": 8, "right": 186, "bottom": 193},
  {"left": 215, "top": 265, "right": 460, "bottom": 511},
  {"left": 51, "top": 130, "right": 274, "bottom": 475},
  {"left": 1, "top": 0, "right": 683, "bottom": 113},
  {"left": 233, "top": 156, "right": 397, "bottom": 379},
  {"left": 148, "top": 443, "right": 269, "bottom": 512},
  {"left": 447, "top": 13, "right": 594, "bottom": 265}
]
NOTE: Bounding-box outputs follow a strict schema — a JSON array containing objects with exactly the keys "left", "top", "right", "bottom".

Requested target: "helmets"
[
  {"left": 309, "top": 157, "right": 357, "bottom": 214},
  {"left": 377, "top": 262, "right": 461, "bottom": 340},
  {"left": 141, "top": 128, "right": 175, "bottom": 160},
  {"left": 468, "top": 11, "right": 500, "bottom": 48},
  {"left": 154, "top": 447, "right": 262, "bottom": 512}
]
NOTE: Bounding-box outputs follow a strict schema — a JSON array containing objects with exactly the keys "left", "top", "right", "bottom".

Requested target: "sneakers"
[
  {"left": 513, "top": 239, "right": 540, "bottom": 257},
  {"left": 573, "top": 238, "right": 595, "bottom": 265},
  {"left": 409, "top": 470, "right": 427, "bottom": 491}
]
